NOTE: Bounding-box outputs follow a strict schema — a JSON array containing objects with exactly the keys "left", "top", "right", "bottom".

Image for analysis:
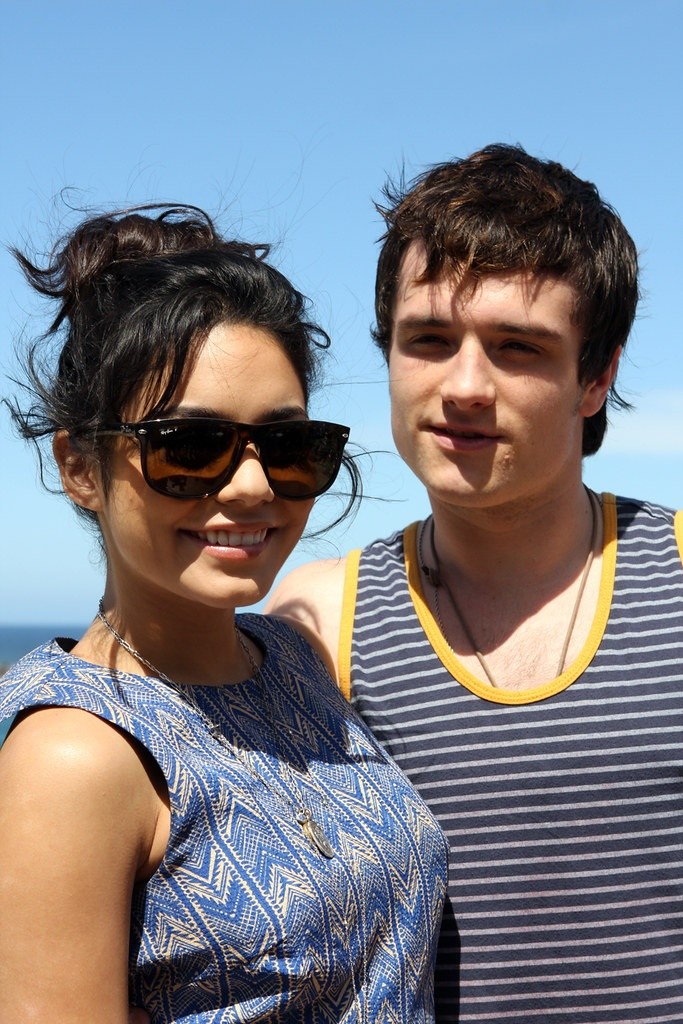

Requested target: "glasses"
[{"left": 84, "top": 414, "right": 350, "bottom": 504}]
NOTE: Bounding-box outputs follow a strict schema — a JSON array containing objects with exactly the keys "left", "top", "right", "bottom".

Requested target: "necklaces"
[
  {"left": 412, "top": 481, "right": 607, "bottom": 690},
  {"left": 96, "top": 598, "right": 335, "bottom": 859}
]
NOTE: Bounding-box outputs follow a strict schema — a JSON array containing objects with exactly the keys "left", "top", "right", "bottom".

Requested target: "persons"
[
  {"left": 267, "top": 142, "right": 683, "bottom": 1024},
  {"left": 0, "top": 204, "right": 452, "bottom": 1024}
]
